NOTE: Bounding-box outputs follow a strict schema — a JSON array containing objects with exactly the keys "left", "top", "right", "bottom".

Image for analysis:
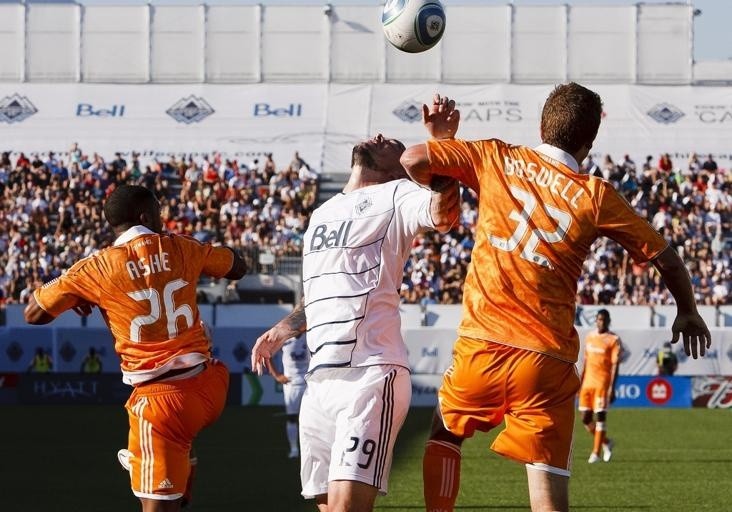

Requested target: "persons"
[
  {"left": 394, "top": 183, "right": 479, "bottom": 304},
  {"left": 576, "top": 309, "right": 623, "bottom": 466},
  {"left": 280, "top": 331, "right": 313, "bottom": 463},
  {"left": 399, "top": 76, "right": 715, "bottom": 512},
  {"left": 655, "top": 340, "right": 679, "bottom": 375},
  {"left": 250, "top": 92, "right": 465, "bottom": 512},
  {"left": 1, "top": 144, "right": 320, "bottom": 307},
  {"left": 24, "top": 179, "right": 246, "bottom": 512},
  {"left": 27, "top": 345, "right": 54, "bottom": 374},
  {"left": 79, "top": 345, "right": 102, "bottom": 375},
  {"left": 572, "top": 152, "right": 731, "bottom": 306}
]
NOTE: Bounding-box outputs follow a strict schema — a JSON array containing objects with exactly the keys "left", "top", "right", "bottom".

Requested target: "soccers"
[{"left": 382, "top": 0, "right": 445, "bottom": 53}]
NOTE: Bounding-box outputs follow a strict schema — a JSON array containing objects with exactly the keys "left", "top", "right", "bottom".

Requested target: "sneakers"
[
  {"left": 602, "top": 437, "right": 616, "bottom": 464},
  {"left": 586, "top": 452, "right": 601, "bottom": 465}
]
